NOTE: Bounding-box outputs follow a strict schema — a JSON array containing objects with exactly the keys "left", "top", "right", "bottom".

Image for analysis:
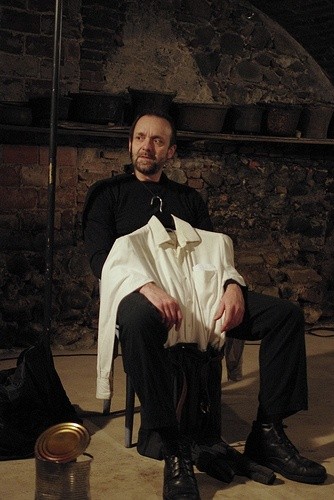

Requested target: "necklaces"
[{"left": 130, "top": 169, "right": 168, "bottom": 199}]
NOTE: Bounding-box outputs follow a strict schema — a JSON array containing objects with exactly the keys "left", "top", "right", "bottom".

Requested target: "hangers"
[{"left": 151, "top": 195, "right": 176, "bottom": 230}]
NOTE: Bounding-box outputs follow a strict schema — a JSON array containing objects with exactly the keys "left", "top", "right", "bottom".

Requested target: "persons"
[{"left": 80, "top": 109, "right": 326, "bottom": 500}]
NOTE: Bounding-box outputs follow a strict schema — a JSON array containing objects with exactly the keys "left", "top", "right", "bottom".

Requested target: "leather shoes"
[
  {"left": 162, "top": 435, "right": 200, "bottom": 500},
  {"left": 244, "top": 420, "right": 327, "bottom": 485}
]
